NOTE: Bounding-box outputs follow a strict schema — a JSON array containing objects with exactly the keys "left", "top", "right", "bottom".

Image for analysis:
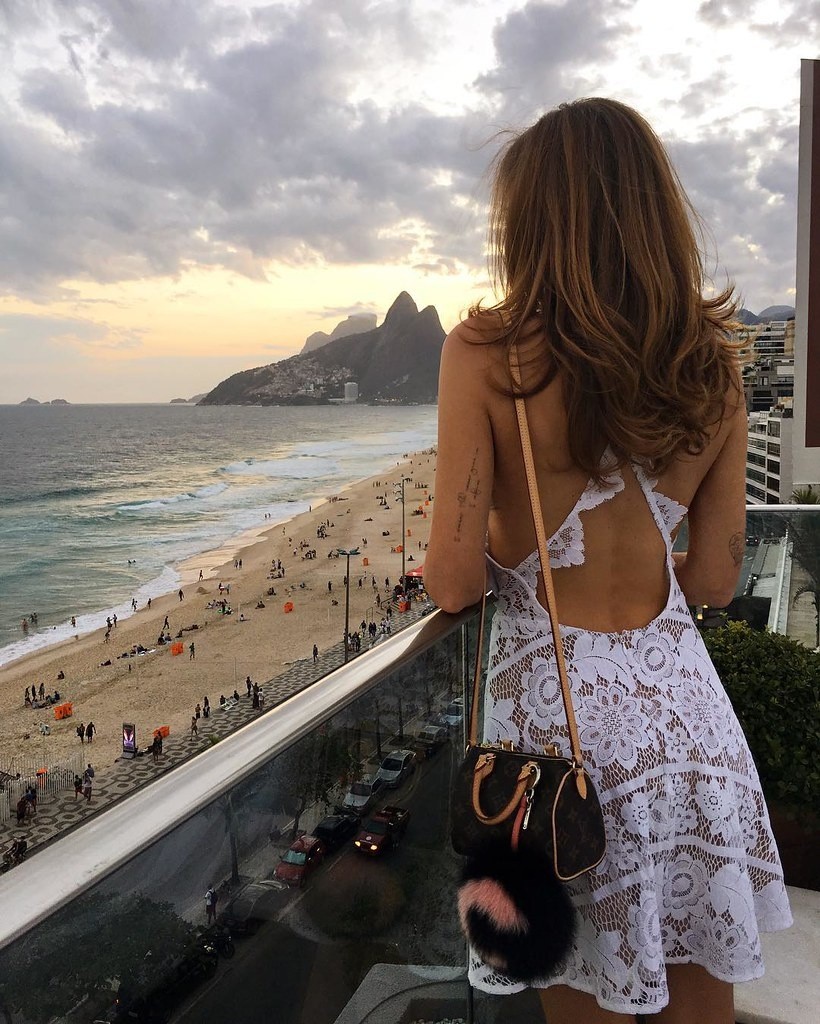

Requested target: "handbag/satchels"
[{"left": 451, "top": 739, "right": 607, "bottom": 881}]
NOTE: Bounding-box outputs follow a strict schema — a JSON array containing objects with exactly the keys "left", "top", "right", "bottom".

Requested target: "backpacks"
[{"left": 209, "top": 890, "right": 218, "bottom": 904}]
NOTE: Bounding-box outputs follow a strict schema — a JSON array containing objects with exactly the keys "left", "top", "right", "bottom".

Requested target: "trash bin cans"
[
  {"left": 170, "top": 642, "right": 184, "bottom": 656},
  {"left": 396, "top": 544, "right": 403, "bottom": 552},
  {"left": 37, "top": 768, "right": 48, "bottom": 786},
  {"left": 363, "top": 558, "right": 368, "bottom": 566},
  {"left": 54, "top": 702, "right": 73, "bottom": 720},
  {"left": 399, "top": 601, "right": 406, "bottom": 612},
  {"left": 153, "top": 725, "right": 170, "bottom": 738},
  {"left": 284, "top": 601, "right": 293, "bottom": 613}
]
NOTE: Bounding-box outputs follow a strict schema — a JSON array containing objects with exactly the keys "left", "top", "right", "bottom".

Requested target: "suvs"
[
  {"left": 114, "top": 943, "right": 219, "bottom": 1023},
  {"left": 221, "top": 879, "right": 293, "bottom": 939}
]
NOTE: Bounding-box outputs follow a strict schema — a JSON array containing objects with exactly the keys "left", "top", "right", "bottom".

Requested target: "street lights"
[
  {"left": 393, "top": 476, "right": 411, "bottom": 595},
  {"left": 336, "top": 547, "right": 361, "bottom": 663}
]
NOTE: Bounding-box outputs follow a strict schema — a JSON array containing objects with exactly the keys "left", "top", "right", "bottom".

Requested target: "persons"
[
  {"left": 86, "top": 721, "right": 97, "bottom": 744},
  {"left": 203, "top": 882, "right": 219, "bottom": 926},
  {"left": 28, "top": 786, "right": 38, "bottom": 812},
  {"left": 146, "top": 675, "right": 266, "bottom": 765},
  {"left": 19, "top": 446, "right": 450, "bottom": 664},
  {"left": 5, "top": 835, "right": 28, "bottom": 866},
  {"left": 83, "top": 763, "right": 94, "bottom": 782},
  {"left": 22, "top": 788, "right": 32, "bottom": 817},
  {"left": 83, "top": 773, "right": 93, "bottom": 803},
  {"left": 73, "top": 775, "right": 85, "bottom": 800},
  {"left": 421, "top": 96, "right": 795, "bottom": 1024},
  {"left": 23, "top": 671, "right": 65, "bottom": 736},
  {"left": 76, "top": 723, "right": 85, "bottom": 745},
  {"left": 15, "top": 796, "right": 27, "bottom": 827},
  {"left": 7, "top": 776, "right": 14, "bottom": 800}
]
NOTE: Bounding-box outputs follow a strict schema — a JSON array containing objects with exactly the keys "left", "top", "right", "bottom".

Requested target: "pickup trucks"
[{"left": 355, "top": 805, "right": 416, "bottom": 860}]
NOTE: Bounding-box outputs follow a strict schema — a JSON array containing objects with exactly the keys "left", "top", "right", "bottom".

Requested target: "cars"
[
  {"left": 315, "top": 810, "right": 360, "bottom": 855},
  {"left": 415, "top": 724, "right": 450, "bottom": 753},
  {"left": 436, "top": 697, "right": 466, "bottom": 730},
  {"left": 374, "top": 748, "right": 418, "bottom": 789},
  {"left": 273, "top": 836, "right": 327, "bottom": 888},
  {"left": 342, "top": 772, "right": 386, "bottom": 815}
]
